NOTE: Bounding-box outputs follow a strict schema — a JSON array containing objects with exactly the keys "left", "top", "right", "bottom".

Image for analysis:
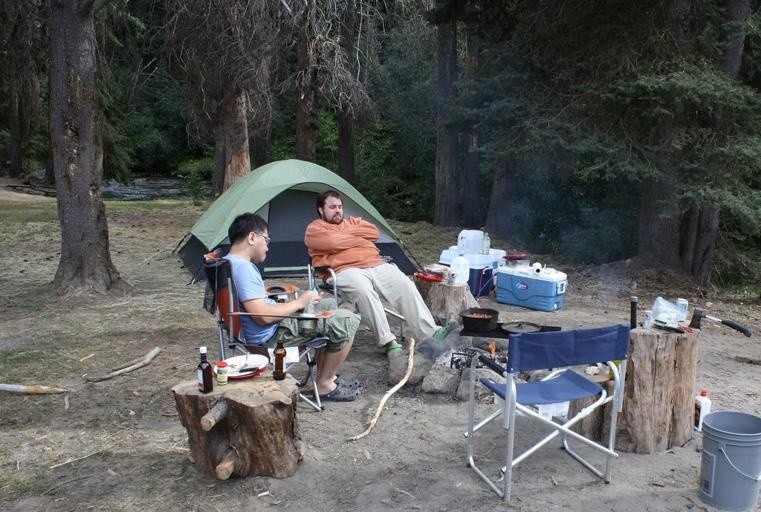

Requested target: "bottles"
[
  {"left": 196, "top": 346, "right": 213, "bottom": 394},
  {"left": 302, "top": 299, "right": 316, "bottom": 334},
  {"left": 271, "top": 340, "right": 287, "bottom": 380},
  {"left": 481, "top": 232, "right": 490, "bottom": 254},
  {"left": 216, "top": 361, "right": 228, "bottom": 385}
]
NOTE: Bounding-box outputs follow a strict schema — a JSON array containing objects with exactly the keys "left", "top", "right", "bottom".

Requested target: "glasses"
[{"left": 254, "top": 231, "right": 270, "bottom": 245}]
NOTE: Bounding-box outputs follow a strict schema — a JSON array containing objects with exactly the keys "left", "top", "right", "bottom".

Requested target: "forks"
[{"left": 242, "top": 352, "right": 249, "bottom": 368}]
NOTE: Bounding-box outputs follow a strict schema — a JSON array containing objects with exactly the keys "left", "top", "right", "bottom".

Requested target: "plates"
[
  {"left": 502, "top": 252, "right": 528, "bottom": 260},
  {"left": 214, "top": 353, "right": 269, "bottom": 377},
  {"left": 413, "top": 270, "right": 454, "bottom": 283}
]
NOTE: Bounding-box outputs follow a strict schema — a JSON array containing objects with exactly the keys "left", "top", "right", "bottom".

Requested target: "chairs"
[
  {"left": 202, "top": 241, "right": 401, "bottom": 411},
  {"left": 467, "top": 324, "right": 631, "bottom": 504}
]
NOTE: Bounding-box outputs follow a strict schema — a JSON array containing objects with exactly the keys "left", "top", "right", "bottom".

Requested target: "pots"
[{"left": 459, "top": 307, "right": 499, "bottom": 331}]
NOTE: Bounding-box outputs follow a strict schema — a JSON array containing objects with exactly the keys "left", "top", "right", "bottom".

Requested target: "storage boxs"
[{"left": 439, "top": 246, "right": 568, "bottom": 312}]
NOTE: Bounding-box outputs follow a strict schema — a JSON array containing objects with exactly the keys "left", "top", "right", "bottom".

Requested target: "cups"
[
  {"left": 518, "top": 258, "right": 530, "bottom": 274},
  {"left": 677, "top": 298, "right": 689, "bottom": 321},
  {"left": 642, "top": 310, "right": 654, "bottom": 330}
]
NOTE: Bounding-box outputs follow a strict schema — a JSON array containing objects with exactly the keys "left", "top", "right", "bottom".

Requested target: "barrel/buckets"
[
  {"left": 697, "top": 411, "right": 761, "bottom": 512},
  {"left": 457, "top": 229, "right": 484, "bottom": 255}
]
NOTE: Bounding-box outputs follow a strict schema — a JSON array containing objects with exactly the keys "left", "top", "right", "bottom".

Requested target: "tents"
[{"left": 177, "top": 158, "right": 427, "bottom": 287}]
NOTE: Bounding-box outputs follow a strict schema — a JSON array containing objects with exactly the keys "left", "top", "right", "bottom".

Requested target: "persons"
[
  {"left": 220, "top": 213, "right": 362, "bottom": 402},
  {"left": 303, "top": 190, "right": 461, "bottom": 360}
]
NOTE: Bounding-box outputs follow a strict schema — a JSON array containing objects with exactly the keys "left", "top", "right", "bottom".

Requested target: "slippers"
[
  {"left": 434, "top": 319, "right": 459, "bottom": 342},
  {"left": 385, "top": 344, "right": 402, "bottom": 355},
  {"left": 313, "top": 374, "right": 361, "bottom": 401}
]
